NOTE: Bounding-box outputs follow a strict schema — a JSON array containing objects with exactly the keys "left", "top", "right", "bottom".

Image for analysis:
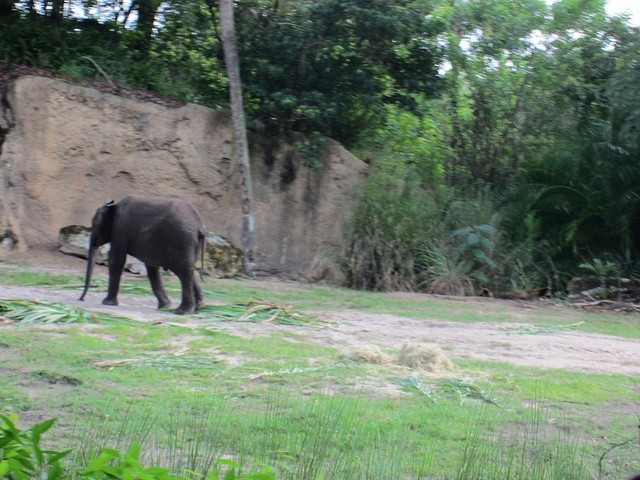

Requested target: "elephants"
[{"left": 78, "top": 195, "right": 206, "bottom": 314}]
